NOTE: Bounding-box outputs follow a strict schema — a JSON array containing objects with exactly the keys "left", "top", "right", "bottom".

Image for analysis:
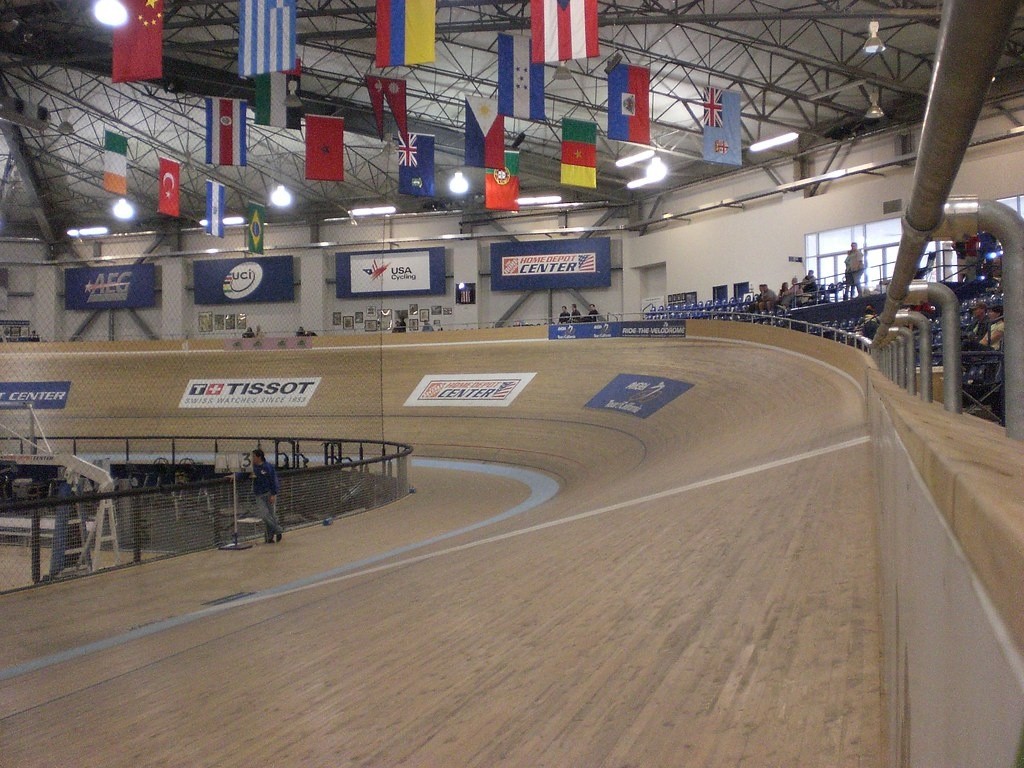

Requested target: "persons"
[
  {"left": 392, "top": 320, "right": 405, "bottom": 332},
  {"left": 570, "top": 304, "right": 581, "bottom": 323},
  {"left": 588, "top": 304, "right": 598, "bottom": 322},
  {"left": 241, "top": 327, "right": 255, "bottom": 338},
  {"left": 558, "top": 306, "right": 570, "bottom": 324},
  {"left": 399, "top": 315, "right": 407, "bottom": 326},
  {"left": 421, "top": 320, "right": 434, "bottom": 332},
  {"left": 251, "top": 449, "right": 284, "bottom": 545},
  {"left": 298, "top": 326, "right": 319, "bottom": 337},
  {"left": 739, "top": 226, "right": 1012, "bottom": 393}
]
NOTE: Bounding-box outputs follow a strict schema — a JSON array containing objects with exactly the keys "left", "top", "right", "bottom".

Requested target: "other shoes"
[
  {"left": 277, "top": 534, "right": 282, "bottom": 541},
  {"left": 266, "top": 539, "right": 274, "bottom": 543}
]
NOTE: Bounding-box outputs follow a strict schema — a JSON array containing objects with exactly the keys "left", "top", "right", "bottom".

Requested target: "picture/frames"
[
  {"left": 431, "top": 306, "right": 441, "bottom": 315},
  {"left": 409, "top": 319, "right": 418, "bottom": 331},
  {"left": 198, "top": 311, "right": 214, "bottom": 332},
  {"left": 408, "top": 304, "right": 418, "bottom": 315},
  {"left": 333, "top": 311, "right": 341, "bottom": 324},
  {"left": 433, "top": 320, "right": 440, "bottom": 325},
  {"left": 226, "top": 313, "right": 236, "bottom": 329},
  {"left": 354, "top": 311, "right": 363, "bottom": 323},
  {"left": 366, "top": 306, "right": 375, "bottom": 316},
  {"left": 343, "top": 315, "right": 354, "bottom": 329},
  {"left": 376, "top": 308, "right": 391, "bottom": 331},
  {"left": 443, "top": 307, "right": 453, "bottom": 315},
  {"left": 237, "top": 313, "right": 247, "bottom": 329},
  {"left": 420, "top": 308, "right": 429, "bottom": 322},
  {"left": 364, "top": 320, "right": 377, "bottom": 330},
  {"left": 214, "top": 315, "right": 225, "bottom": 330}
]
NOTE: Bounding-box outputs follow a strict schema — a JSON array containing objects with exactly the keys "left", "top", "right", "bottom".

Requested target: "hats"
[
  {"left": 969, "top": 302, "right": 987, "bottom": 309},
  {"left": 866, "top": 306, "right": 872, "bottom": 310}
]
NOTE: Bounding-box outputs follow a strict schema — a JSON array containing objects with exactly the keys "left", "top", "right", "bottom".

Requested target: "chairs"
[
  {"left": 808, "top": 280, "right": 1006, "bottom": 423},
  {"left": 646, "top": 294, "right": 764, "bottom": 321}
]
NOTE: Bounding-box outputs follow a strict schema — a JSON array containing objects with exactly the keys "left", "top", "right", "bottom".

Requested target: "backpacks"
[{"left": 863, "top": 317, "right": 880, "bottom": 340}]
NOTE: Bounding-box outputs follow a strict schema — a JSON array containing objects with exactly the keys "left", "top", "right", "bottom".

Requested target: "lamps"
[
  {"left": 864, "top": 80, "right": 883, "bottom": 118},
  {"left": 449, "top": 89, "right": 469, "bottom": 193},
  {"left": 554, "top": 58, "right": 571, "bottom": 81},
  {"left": 863, "top": 16, "right": 886, "bottom": 53},
  {"left": 271, "top": 135, "right": 292, "bottom": 207},
  {"left": 646, "top": 93, "right": 667, "bottom": 181},
  {"left": 57, "top": 68, "right": 74, "bottom": 132},
  {"left": 285, "top": 78, "right": 302, "bottom": 107}
]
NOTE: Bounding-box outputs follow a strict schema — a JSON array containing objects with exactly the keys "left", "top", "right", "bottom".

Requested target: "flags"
[
  {"left": 91, "top": 55, "right": 446, "bottom": 262},
  {"left": 238, "top": 0, "right": 296, "bottom": 79},
  {"left": 375, "top": 0, "right": 438, "bottom": 69},
  {"left": 560, "top": 116, "right": 599, "bottom": 189},
  {"left": 111, "top": 0, "right": 163, "bottom": 86},
  {"left": 529, "top": 0, "right": 598, "bottom": 65},
  {"left": 462, "top": 93, "right": 505, "bottom": 170},
  {"left": 603, "top": 58, "right": 652, "bottom": 146},
  {"left": 699, "top": 85, "right": 743, "bottom": 167},
  {"left": 495, "top": 30, "right": 549, "bottom": 122}
]
